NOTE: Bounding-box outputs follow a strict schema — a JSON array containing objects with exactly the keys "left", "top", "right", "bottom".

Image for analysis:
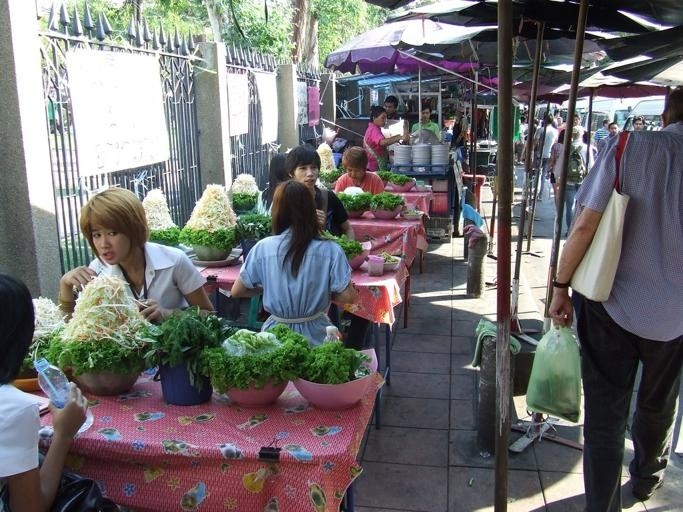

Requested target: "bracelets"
[
  {"left": 553, "top": 280, "right": 571, "bottom": 288},
  {"left": 60, "top": 298, "right": 74, "bottom": 314}
]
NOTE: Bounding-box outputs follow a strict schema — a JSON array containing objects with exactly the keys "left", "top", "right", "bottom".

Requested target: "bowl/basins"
[
  {"left": 370, "top": 205, "right": 401, "bottom": 220},
  {"left": 347, "top": 241, "right": 373, "bottom": 270},
  {"left": 192, "top": 244, "right": 232, "bottom": 261},
  {"left": 74, "top": 368, "right": 143, "bottom": 395},
  {"left": 225, "top": 375, "right": 290, "bottom": 406},
  {"left": 346, "top": 208, "right": 363, "bottom": 219},
  {"left": 416, "top": 184, "right": 433, "bottom": 192},
  {"left": 403, "top": 211, "right": 424, "bottom": 221},
  {"left": 292, "top": 346, "right": 379, "bottom": 411},
  {"left": 381, "top": 258, "right": 399, "bottom": 271},
  {"left": 380, "top": 177, "right": 416, "bottom": 194}
]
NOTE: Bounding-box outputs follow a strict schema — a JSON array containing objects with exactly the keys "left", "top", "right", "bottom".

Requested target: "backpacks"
[{"left": 567, "top": 144, "right": 586, "bottom": 182}]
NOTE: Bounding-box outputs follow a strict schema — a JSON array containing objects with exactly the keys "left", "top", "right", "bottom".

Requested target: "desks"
[
  {"left": 348, "top": 209, "right": 428, "bottom": 327},
  {"left": 178, "top": 243, "right": 409, "bottom": 428},
  {"left": 328, "top": 180, "right": 436, "bottom": 261},
  {"left": 23, "top": 371, "right": 381, "bottom": 511}
]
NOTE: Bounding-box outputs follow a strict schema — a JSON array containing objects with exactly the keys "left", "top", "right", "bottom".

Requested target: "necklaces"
[{"left": 114, "top": 258, "right": 146, "bottom": 303}]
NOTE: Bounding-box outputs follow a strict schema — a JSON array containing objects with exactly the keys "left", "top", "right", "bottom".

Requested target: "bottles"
[
  {"left": 399, "top": 192, "right": 407, "bottom": 216},
  {"left": 32, "top": 357, "right": 93, "bottom": 435},
  {"left": 322, "top": 325, "right": 339, "bottom": 346}
]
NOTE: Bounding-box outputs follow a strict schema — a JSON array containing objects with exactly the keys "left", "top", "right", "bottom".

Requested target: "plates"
[
  {"left": 393, "top": 144, "right": 412, "bottom": 171},
  {"left": 411, "top": 145, "right": 431, "bottom": 171},
  {"left": 13, "top": 377, "right": 39, "bottom": 393},
  {"left": 189, "top": 253, "right": 236, "bottom": 266},
  {"left": 430, "top": 145, "right": 449, "bottom": 172}
]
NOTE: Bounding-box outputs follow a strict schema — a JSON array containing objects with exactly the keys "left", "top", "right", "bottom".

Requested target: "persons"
[
  {"left": 288, "top": 145, "right": 356, "bottom": 243},
  {"left": 364, "top": 93, "right": 487, "bottom": 172},
  {"left": 335, "top": 147, "right": 383, "bottom": 195},
  {"left": 549, "top": 86, "right": 683, "bottom": 512},
  {"left": 56, "top": 186, "right": 216, "bottom": 323},
  {"left": 229, "top": 180, "right": 359, "bottom": 350},
  {"left": 259, "top": 150, "right": 292, "bottom": 217},
  {"left": 0, "top": 274, "right": 114, "bottom": 512},
  {"left": 519, "top": 109, "right": 645, "bottom": 237}
]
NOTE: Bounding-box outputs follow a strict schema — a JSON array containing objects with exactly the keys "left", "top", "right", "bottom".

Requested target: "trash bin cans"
[{"left": 469, "top": 149, "right": 490, "bottom": 166}]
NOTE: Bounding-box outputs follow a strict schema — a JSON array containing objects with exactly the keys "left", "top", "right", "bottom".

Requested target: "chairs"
[
  {"left": 247, "top": 292, "right": 271, "bottom": 331},
  {"left": 47, "top": 477, "right": 109, "bottom": 511},
  {"left": 478, "top": 153, "right": 496, "bottom": 184},
  {"left": 205, "top": 276, "right": 241, "bottom": 321}
]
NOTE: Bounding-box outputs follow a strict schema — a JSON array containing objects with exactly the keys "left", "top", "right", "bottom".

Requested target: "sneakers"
[{"left": 632, "top": 476, "right": 665, "bottom": 498}]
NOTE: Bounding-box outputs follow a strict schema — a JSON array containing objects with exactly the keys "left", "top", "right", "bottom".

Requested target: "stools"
[{"left": 482, "top": 318, "right": 585, "bottom": 453}]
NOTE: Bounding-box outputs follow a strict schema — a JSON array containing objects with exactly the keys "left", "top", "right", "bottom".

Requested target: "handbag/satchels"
[
  {"left": 570, "top": 187, "right": 630, "bottom": 302},
  {"left": 53, "top": 471, "right": 120, "bottom": 511}
]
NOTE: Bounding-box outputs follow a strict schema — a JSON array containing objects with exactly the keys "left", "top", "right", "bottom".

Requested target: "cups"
[
  {"left": 415, "top": 181, "right": 425, "bottom": 185},
  {"left": 367, "top": 258, "right": 383, "bottom": 276}
]
